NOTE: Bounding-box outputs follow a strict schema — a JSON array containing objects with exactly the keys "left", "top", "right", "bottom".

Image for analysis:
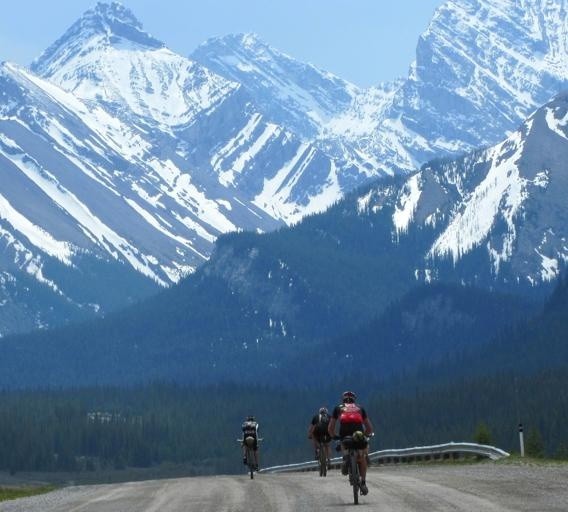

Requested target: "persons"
[
  {"left": 328, "top": 392, "right": 372, "bottom": 495},
  {"left": 240, "top": 416, "right": 259, "bottom": 471},
  {"left": 309, "top": 408, "right": 332, "bottom": 470}
]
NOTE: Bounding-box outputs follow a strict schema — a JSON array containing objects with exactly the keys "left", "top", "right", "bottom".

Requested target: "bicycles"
[
  {"left": 308, "top": 430, "right": 376, "bottom": 503},
  {"left": 236, "top": 434, "right": 265, "bottom": 480}
]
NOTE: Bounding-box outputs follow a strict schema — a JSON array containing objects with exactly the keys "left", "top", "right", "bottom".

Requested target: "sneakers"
[
  {"left": 342, "top": 458, "right": 350, "bottom": 476},
  {"left": 255, "top": 466, "right": 259, "bottom": 472},
  {"left": 359, "top": 480, "right": 368, "bottom": 495},
  {"left": 243, "top": 456, "right": 247, "bottom": 463},
  {"left": 314, "top": 455, "right": 320, "bottom": 460}
]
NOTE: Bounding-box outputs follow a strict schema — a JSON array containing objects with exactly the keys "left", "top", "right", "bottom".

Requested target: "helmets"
[
  {"left": 319, "top": 407, "right": 329, "bottom": 414},
  {"left": 246, "top": 415, "right": 256, "bottom": 421},
  {"left": 343, "top": 391, "right": 358, "bottom": 401}
]
